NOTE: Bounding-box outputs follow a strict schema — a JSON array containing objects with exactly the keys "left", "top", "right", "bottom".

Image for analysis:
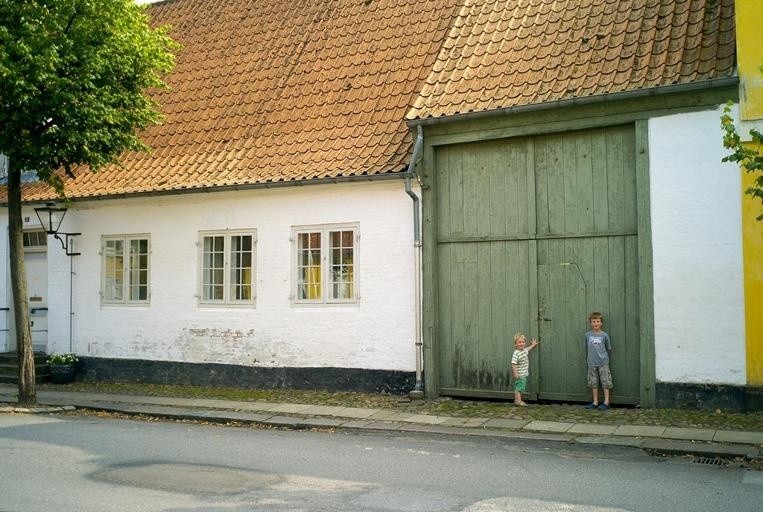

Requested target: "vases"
[{"left": 50, "top": 364, "right": 77, "bottom": 384}]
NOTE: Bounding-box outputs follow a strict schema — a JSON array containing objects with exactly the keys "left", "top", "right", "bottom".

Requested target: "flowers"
[{"left": 46, "top": 353, "right": 79, "bottom": 366}]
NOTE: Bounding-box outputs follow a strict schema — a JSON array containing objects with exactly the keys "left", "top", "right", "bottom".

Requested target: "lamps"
[{"left": 34, "top": 201, "right": 81, "bottom": 256}]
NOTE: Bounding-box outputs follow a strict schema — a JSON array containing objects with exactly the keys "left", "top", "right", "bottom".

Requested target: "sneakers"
[{"left": 514, "top": 400, "right": 529, "bottom": 407}]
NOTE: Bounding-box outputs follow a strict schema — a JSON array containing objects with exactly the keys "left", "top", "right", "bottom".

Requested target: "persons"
[
  {"left": 511, "top": 332, "right": 539, "bottom": 406},
  {"left": 584, "top": 311, "right": 612, "bottom": 411}
]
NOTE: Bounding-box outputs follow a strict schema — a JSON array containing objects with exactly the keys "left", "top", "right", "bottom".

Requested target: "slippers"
[
  {"left": 584, "top": 403, "right": 599, "bottom": 409},
  {"left": 598, "top": 403, "right": 611, "bottom": 410}
]
